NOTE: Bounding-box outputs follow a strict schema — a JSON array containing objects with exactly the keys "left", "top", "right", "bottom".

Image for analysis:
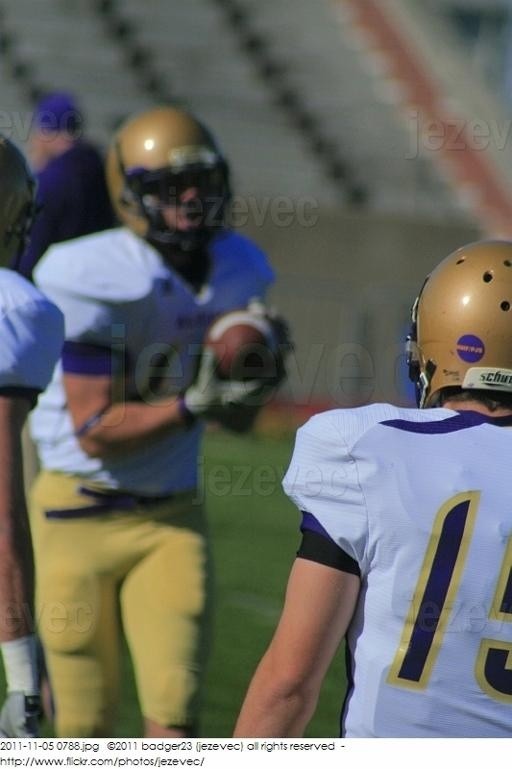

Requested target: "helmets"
[
  {"left": 106, "top": 106, "right": 232, "bottom": 252},
  {"left": 406, "top": 240, "right": 512, "bottom": 409}
]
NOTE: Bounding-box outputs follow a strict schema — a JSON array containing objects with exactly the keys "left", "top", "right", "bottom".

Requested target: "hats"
[{"left": 34, "top": 98, "right": 81, "bottom": 130}]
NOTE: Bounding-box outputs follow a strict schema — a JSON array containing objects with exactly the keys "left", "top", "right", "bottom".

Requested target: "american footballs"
[{"left": 208, "top": 313, "right": 273, "bottom": 433}]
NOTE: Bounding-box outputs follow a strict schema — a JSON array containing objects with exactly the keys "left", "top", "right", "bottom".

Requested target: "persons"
[
  {"left": 0, "top": 129, "right": 65, "bottom": 738},
  {"left": 26, "top": 104, "right": 294, "bottom": 737},
  {"left": 12, "top": 90, "right": 119, "bottom": 285},
  {"left": 232, "top": 240, "right": 512, "bottom": 738}
]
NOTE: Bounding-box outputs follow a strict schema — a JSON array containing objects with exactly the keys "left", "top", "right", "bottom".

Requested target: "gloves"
[
  {"left": 247, "top": 301, "right": 296, "bottom": 357},
  {"left": 178, "top": 346, "right": 276, "bottom": 431},
  {"left": 1, "top": 691, "right": 46, "bottom": 738}
]
